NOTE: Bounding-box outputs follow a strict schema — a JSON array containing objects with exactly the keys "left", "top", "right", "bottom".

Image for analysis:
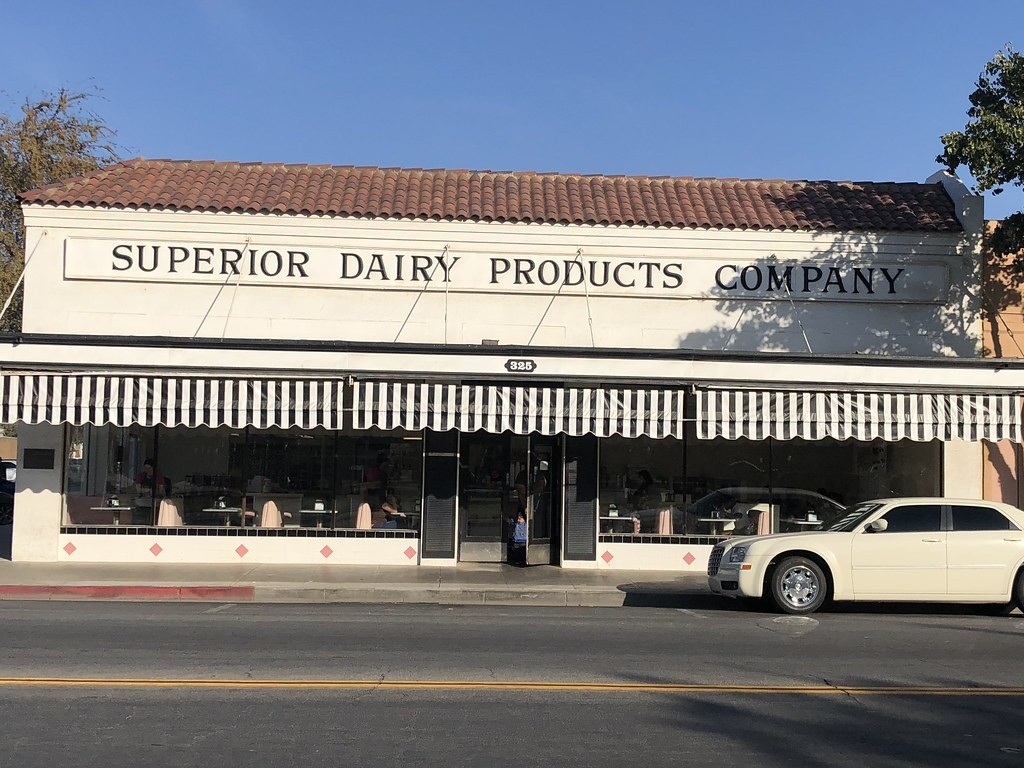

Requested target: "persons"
[
  {"left": 373, "top": 494, "right": 398, "bottom": 529},
  {"left": 627, "top": 469, "right": 659, "bottom": 510},
  {"left": 718, "top": 499, "right": 732, "bottom": 518},
  {"left": 136, "top": 459, "right": 164, "bottom": 496},
  {"left": 508, "top": 458, "right": 547, "bottom": 564}
]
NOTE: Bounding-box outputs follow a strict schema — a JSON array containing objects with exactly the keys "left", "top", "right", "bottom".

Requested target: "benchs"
[
  {"left": 638, "top": 508, "right": 674, "bottom": 536},
  {"left": 748, "top": 510, "right": 770, "bottom": 534},
  {"left": 62, "top": 496, "right": 132, "bottom": 525},
  {"left": 156, "top": 498, "right": 188, "bottom": 526},
  {"left": 260, "top": 500, "right": 292, "bottom": 528},
  {"left": 356, "top": 501, "right": 373, "bottom": 530}
]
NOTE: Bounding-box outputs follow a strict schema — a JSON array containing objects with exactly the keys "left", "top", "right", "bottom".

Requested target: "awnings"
[
  {"left": 0, "top": 371, "right": 344, "bottom": 430},
  {"left": 352, "top": 381, "right": 685, "bottom": 444},
  {"left": 694, "top": 389, "right": 1023, "bottom": 444}
]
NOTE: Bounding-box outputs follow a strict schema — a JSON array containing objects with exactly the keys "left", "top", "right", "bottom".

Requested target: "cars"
[
  {"left": 706, "top": 496, "right": 1024, "bottom": 615},
  {"left": 618, "top": 487, "right": 849, "bottom": 532}
]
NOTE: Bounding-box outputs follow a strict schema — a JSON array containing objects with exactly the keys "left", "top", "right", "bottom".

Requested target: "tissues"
[
  {"left": 804, "top": 510, "right": 818, "bottom": 522},
  {"left": 107, "top": 495, "right": 120, "bottom": 508},
  {"left": 608, "top": 503, "right": 619, "bottom": 517},
  {"left": 314, "top": 499, "right": 325, "bottom": 511},
  {"left": 216, "top": 496, "right": 227, "bottom": 509}
]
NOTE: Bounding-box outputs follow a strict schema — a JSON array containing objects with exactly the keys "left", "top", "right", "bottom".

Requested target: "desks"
[
  {"left": 698, "top": 518, "right": 735, "bottom": 535},
  {"left": 391, "top": 511, "right": 419, "bottom": 530},
  {"left": 781, "top": 519, "right": 823, "bottom": 532},
  {"left": 299, "top": 509, "right": 338, "bottom": 527},
  {"left": 600, "top": 516, "right": 634, "bottom": 533},
  {"left": 203, "top": 508, "right": 241, "bottom": 526},
  {"left": 90, "top": 506, "right": 135, "bottom": 524}
]
